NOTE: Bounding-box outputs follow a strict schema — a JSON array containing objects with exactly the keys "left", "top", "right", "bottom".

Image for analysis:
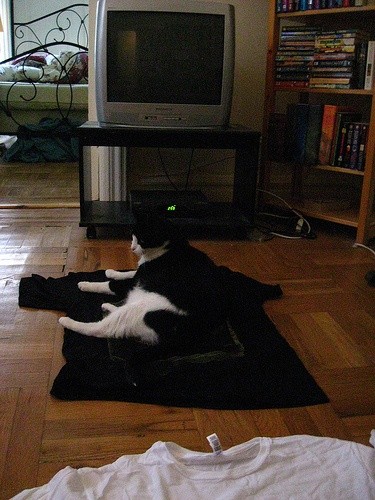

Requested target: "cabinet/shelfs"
[
  {"left": 258, "top": 0, "right": 375, "bottom": 249},
  {"left": 76, "top": 118, "right": 263, "bottom": 243}
]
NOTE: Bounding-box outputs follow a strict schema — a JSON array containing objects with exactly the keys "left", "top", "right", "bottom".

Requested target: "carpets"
[{"left": 18, "top": 239, "right": 333, "bottom": 410}]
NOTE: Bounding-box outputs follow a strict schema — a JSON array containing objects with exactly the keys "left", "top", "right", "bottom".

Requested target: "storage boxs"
[{"left": 302, "top": 197, "right": 352, "bottom": 213}]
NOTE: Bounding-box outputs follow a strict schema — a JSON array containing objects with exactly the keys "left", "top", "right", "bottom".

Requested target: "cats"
[{"left": 58, "top": 203, "right": 208, "bottom": 344}]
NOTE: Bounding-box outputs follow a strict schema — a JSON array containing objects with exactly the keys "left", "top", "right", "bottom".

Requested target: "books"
[
  {"left": 265, "top": 103, "right": 369, "bottom": 171},
  {"left": 277, "top": 0, "right": 375, "bottom": 13},
  {"left": 271, "top": 26, "right": 375, "bottom": 90}
]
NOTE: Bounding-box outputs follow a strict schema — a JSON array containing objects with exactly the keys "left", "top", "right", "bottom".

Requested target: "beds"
[{"left": 0, "top": 3, "right": 89, "bottom": 137}]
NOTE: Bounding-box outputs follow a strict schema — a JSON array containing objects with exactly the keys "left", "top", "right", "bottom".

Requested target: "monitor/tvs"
[{"left": 86, "top": 0, "right": 236, "bottom": 128}]
